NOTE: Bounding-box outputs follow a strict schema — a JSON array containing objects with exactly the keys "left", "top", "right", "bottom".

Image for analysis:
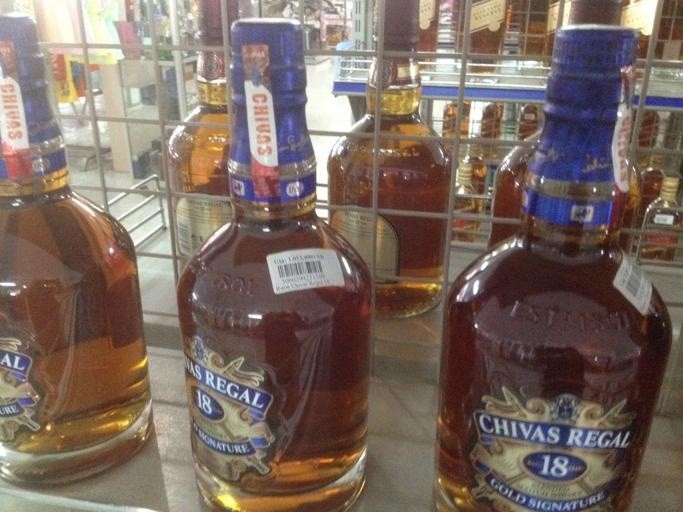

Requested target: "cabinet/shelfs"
[{"left": 0, "top": 0, "right": 683, "bottom": 512}]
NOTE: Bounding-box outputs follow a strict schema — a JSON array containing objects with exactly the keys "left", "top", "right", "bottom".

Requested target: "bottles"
[
  {"left": 327, "top": 2, "right": 452, "bottom": 317},
  {"left": 434, "top": 23, "right": 672, "bottom": 512},
  {"left": 1, "top": 16, "right": 154, "bottom": 489},
  {"left": 165, "top": 0, "right": 372, "bottom": 511}
]
